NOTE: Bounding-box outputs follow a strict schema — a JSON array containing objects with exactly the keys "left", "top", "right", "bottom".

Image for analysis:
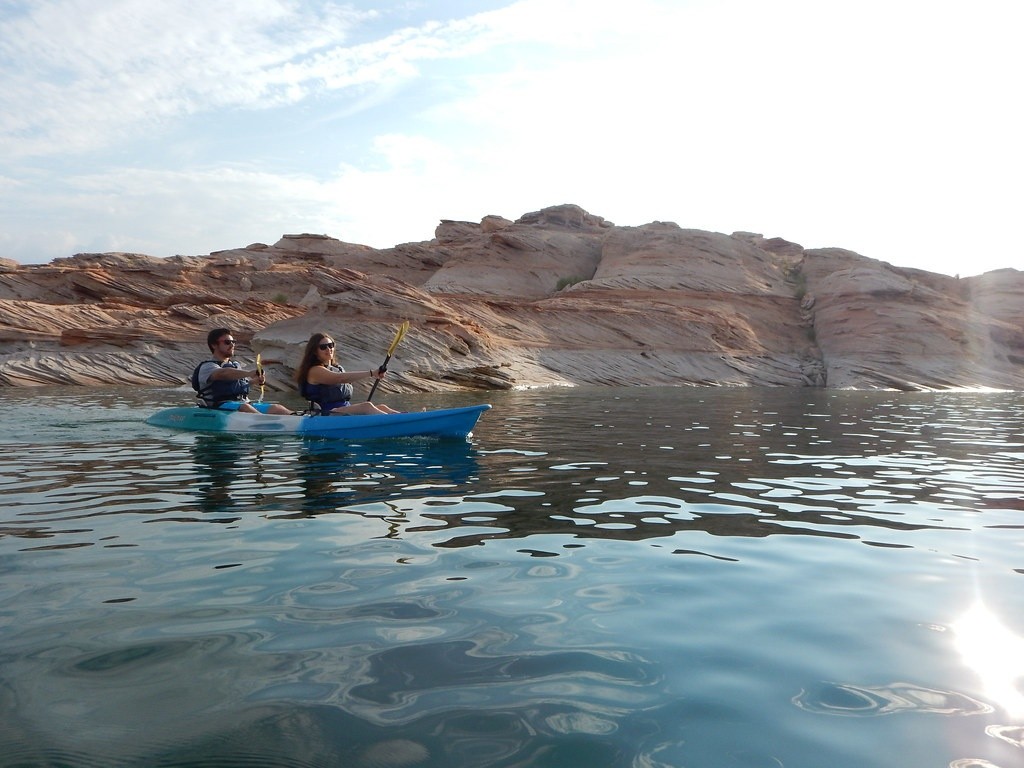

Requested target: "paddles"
[
  {"left": 256, "top": 353, "right": 267, "bottom": 397},
  {"left": 367, "top": 320, "right": 408, "bottom": 401}
]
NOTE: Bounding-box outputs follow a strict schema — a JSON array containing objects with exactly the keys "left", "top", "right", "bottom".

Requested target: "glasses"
[
  {"left": 217, "top": 339, "right": 237, "bottom": 345},
  {"left": 317, "top": 343, "right": 334, "bottom": 350}
]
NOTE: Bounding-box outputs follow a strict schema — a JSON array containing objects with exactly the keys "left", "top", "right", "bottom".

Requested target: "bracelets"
[{"left": 370, "top": 369, "right": 373, "bottom": 377}]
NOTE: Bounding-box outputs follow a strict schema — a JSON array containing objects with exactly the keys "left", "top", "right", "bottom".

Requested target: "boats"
[{"left": 143, "top": 403, "right": 493, "bottom": 440}]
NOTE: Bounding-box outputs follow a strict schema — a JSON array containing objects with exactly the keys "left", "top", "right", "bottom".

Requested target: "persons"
[
  {"left": 295, "top": 332, "right": 427, "bottom": 415},
  {"left": 191, "top": 327, "right": 309, "bottom": 417}
]
